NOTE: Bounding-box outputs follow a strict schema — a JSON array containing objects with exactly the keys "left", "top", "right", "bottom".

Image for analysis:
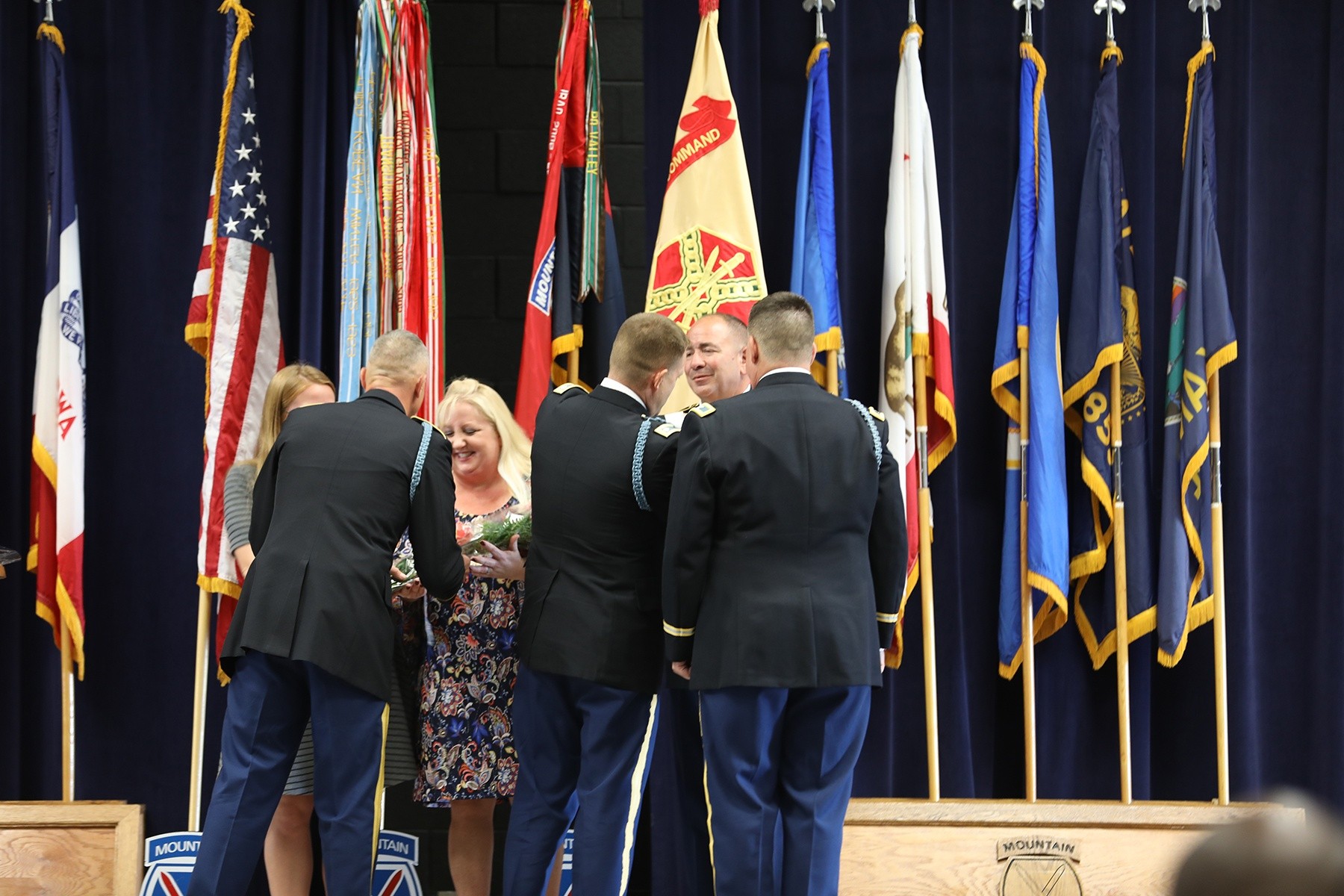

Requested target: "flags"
[
  {"left": 27, "top": 24, "right": 91, "bottom": 681},
  {"left": 182, "top": 0, "right": 1238, "bottom": 686}
]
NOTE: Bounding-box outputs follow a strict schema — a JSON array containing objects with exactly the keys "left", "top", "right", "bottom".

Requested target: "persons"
[
  {"left": 1174, "top": 793, "right": 1344, "bottom": 896},
  {"left": 186, "top": 292, "right": 908, "bottom": 896}
]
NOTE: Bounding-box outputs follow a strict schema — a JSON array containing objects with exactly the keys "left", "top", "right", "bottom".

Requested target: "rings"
[{"left": 486, "top": 567, "right": 489, "bottom": 575}]
